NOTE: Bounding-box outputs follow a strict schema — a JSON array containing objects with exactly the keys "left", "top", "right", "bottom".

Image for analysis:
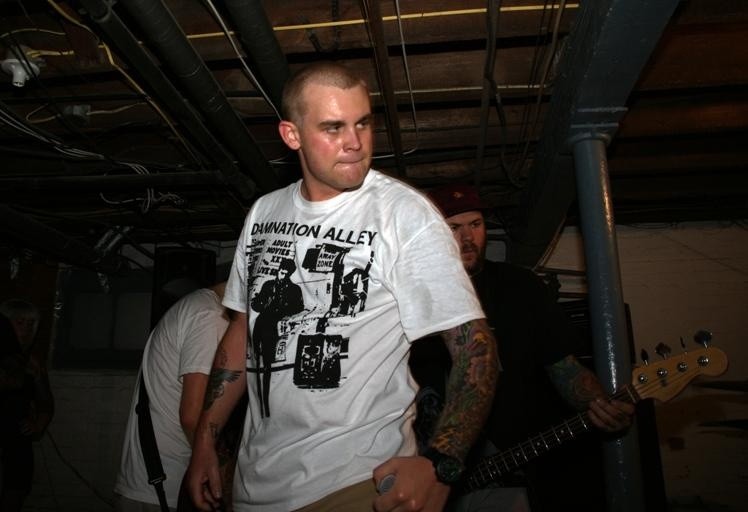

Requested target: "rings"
[{"left": 616, "top": 412, "right": 626, "bottom": 422}]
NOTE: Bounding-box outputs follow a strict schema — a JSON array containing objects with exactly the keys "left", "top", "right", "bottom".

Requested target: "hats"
[{"left": 426, "top": 184, "right": 487, "bottom": 217}]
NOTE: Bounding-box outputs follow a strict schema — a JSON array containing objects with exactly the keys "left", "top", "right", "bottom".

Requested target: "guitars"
[{"left": 411, "top": 329, "right": 728, "bottom": 511}]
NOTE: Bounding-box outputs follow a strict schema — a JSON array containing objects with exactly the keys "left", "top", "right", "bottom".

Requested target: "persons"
[
  {"left": 113, "top": 276, "right": 233, "bottom": 511},
  {"left": 1, "top": 300, "right": 56, "bottom": 511},
  {"left": 184, "top": 61, "right": 500, "bottom": 512},
  {"left": 408, "top": 187, "right": 635, "bottom": 512}
]
